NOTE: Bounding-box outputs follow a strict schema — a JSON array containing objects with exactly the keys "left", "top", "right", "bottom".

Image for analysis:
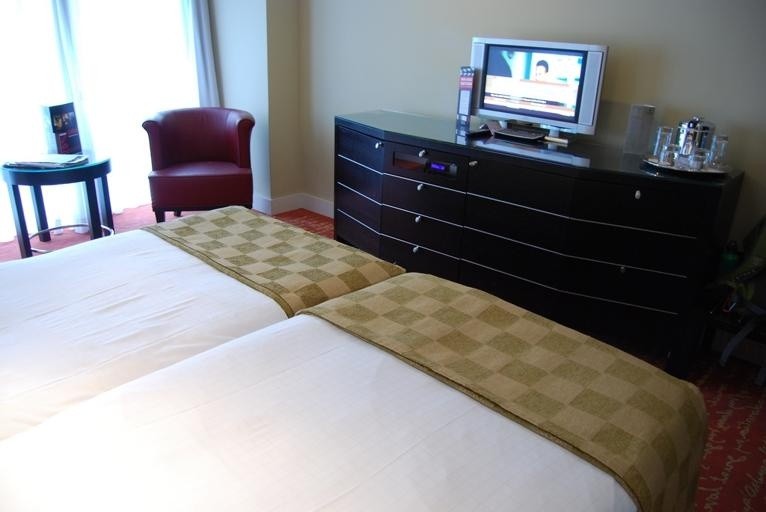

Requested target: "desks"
[{"left": 2, "top": 153, "right": 113, "bottom": 259}]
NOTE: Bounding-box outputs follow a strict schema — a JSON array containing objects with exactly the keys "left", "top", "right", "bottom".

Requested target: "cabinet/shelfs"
[{"left": 329, "top": 109, "right": 744, "bottom": 376}]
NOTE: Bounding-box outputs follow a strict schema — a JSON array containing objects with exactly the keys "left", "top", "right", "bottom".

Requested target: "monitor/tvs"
[{"left": 468, "top": 37, "right": 609, "bottom": 141}]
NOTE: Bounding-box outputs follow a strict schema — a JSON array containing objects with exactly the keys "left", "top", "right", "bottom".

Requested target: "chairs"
[{"left": 140, "top": 106, "right": 256, "bottom": 224}]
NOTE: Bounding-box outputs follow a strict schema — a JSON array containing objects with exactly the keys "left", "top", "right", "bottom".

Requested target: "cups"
[{"left": 653, "top": 127, "right": 728, "bottom": 171}]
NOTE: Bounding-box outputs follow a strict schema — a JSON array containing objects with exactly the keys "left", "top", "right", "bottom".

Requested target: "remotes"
[{"left": 466, "top": 128, "right": 492, "bottom": 140}]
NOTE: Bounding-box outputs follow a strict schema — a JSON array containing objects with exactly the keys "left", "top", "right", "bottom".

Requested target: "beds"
[
  {"left": 0, "top": 205, "right": 408, "bottom": 440},
  {"left": 0, "top": 273, "right": 707, "bottom": 510}
]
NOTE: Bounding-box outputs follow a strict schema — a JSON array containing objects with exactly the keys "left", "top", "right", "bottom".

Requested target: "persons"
[{"left": 536, "top": 60, "right": 548, "bottom": 81}]
